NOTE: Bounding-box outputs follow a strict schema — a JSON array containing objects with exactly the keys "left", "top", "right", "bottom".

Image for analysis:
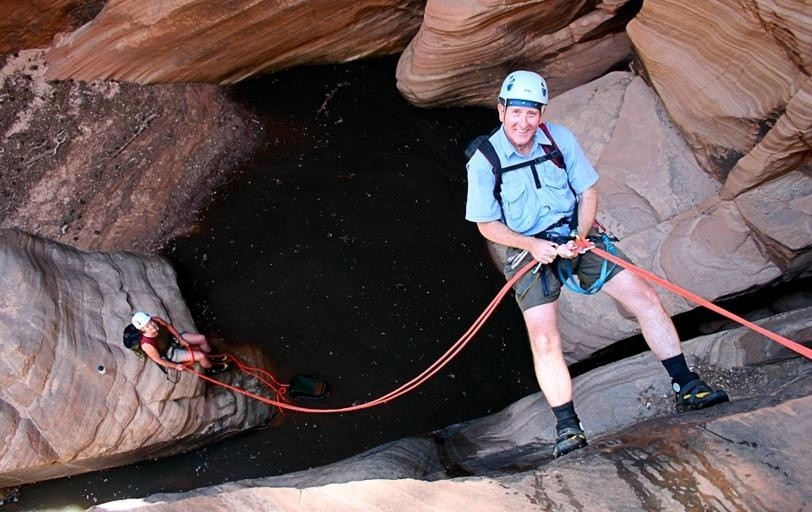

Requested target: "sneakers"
[
  {"left": 670, "top": 371, "right": 730, "bottom": 415},
  {"left": 204, "top": 363, "right": 228, "bottom": 371},
  {"left": 553, "top": 415, "right": 588, "bottom": 458},
  {"left": 211, "top": 354, "right": 227, "bottom": 362}
]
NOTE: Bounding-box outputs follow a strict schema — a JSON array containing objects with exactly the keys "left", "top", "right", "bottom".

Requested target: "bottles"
[{"left": 97, "top": 365, "right": 107, "bottom": 375}]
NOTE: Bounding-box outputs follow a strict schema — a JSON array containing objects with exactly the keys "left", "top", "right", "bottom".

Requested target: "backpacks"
[{"left": 123, "top": 323, "right": 148, "bottom": 360}]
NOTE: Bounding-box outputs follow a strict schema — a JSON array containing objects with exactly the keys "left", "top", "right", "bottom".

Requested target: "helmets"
[
  {"left": 132, "top": 312, "right": 152, "bottom": 331},
  {"left": 497, "top": 70, "right": 550, "bottom": 109}
]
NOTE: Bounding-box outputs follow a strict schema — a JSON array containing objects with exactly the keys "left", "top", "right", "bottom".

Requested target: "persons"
[
  {"left": 132, "top": 312, "right": 229, "bottom": 373},
  {"left": 466, "top": 70, "right": 730, "bottom": 458}
]
